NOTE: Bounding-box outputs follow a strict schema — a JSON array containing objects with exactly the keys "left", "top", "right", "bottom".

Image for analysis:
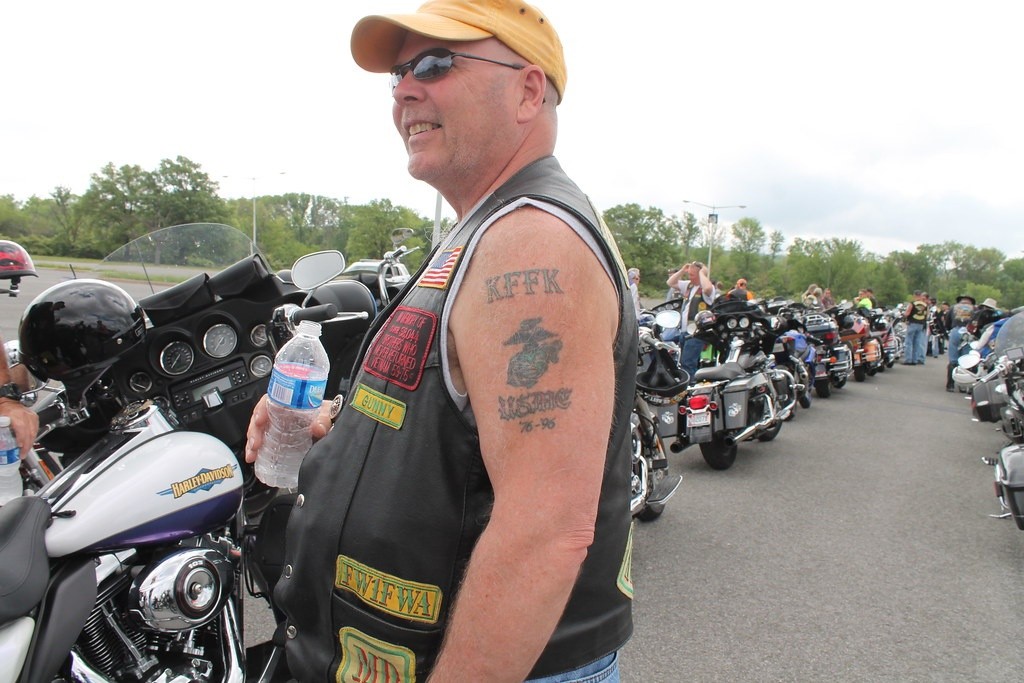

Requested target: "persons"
[
  {"left": 901, "top": 290, "right": 928, "bottom": 365},
  {"left": 854, "top": 289, "right": 876, "bottom": 310},
  {"left": 918, "top": 293, "right": 932, "bottom": 364},
  {"left": 945, "top": 294, "right": 975, "bottom": 330},
  {"left": 667, "top": 261, "right": 715, "bottom": 332},
  {"left": 948, "top": 319, "right": 977, "bottom": 393},
  {"left": 801, "top": 284, "right": 825, "bottom": 310},
  {"left": 727, "top": 279, "right": 752, "bottom": 300},
  {"left": 967, "top": 311, "right": 1012, "bottom": 406},
  {"left": 712, "top": 280, "right": 723, "bottom": 298},
  {"left": 666, "top": 268, "right": 682, "bottom": 298},
  {"left": 929, "top": 298, "right": 949, "bottom": 357},
  {"left": 628, "top": 267, "right": 644, "bottom": 311},
  {"left": 245, "top": 0, "right": 635, "bottom": 683},
  {"left": 973, "top": 298, "right": 999, "bottom": 320},
  {"left": 0, "top": 337, "right": 39, "bottom": 459},
  {"left": 822, "top": 288, "right": 834, "bottom": 308}
]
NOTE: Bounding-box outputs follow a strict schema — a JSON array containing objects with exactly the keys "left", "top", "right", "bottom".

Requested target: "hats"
[
  {"left": 979, "top": 297, "right": 997, "bottom": 310},
  {"left": 349, "top": 0, "right": 568, "bottom": 106},
  {"left": 955, "top": 294, "right": 976, "bottom": 305}
]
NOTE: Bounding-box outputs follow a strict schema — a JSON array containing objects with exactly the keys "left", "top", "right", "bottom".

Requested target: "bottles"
[
  {"left": 0, "top": 417, "right": 24, "bottom": 505},
  {"left": 252, "top": 320, "right": 328, "bottom": 485}
]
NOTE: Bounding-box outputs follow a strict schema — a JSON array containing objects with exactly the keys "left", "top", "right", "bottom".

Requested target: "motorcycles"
[
  {"left": 956, "top": 311, "right": 1024, "bottom": 532},
  {"left": 747, "top": 296, "right": 1024, "bottom": 425},
  {"left": 669, "top": 300, "right": 798, "bottom": 472},
  {"left": 630, "top": 304, "right": 685, "bottom": 524},
  {"left": 0, "top": 222, "right": 377, "bottom": 683}
]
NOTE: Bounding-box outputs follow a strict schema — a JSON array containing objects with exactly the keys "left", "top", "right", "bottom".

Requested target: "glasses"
[
  {"left": 387, "top": 47, "right": 547, "bottom": 105},
  {"left": 739, "top": 282, "right": 747, "bottom": 287}
]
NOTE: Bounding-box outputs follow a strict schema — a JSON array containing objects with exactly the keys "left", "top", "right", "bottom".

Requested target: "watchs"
[{"left": 0, "top": 382, "right": 21, "bottom": 400}]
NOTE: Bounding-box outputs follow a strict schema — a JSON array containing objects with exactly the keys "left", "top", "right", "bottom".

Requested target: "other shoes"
[{"left": 902, "top": 360, "right": 924, "bottom": 366}]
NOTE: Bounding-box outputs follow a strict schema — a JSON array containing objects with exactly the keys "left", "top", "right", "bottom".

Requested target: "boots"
[{"left": 946, "top": 364, "right": 954, "bottom": 392}]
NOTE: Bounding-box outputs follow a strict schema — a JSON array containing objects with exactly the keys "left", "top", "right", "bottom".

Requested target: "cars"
[{"left": 272, "top": 243, "right": 420, "bottom": 315}]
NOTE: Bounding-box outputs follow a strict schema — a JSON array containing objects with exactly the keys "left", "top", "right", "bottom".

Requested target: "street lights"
[
  {"left": 684, "top": 199, "right": 748, "bottom": 280},
  {"left": 222, "top": 171, "right": 286, "bottom": 245}
]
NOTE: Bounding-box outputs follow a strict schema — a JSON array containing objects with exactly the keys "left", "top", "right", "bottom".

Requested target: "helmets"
[
  {"left": 0, "top": 240, "right": 38, "bottom": 280},
  {"left": 16, "top": 279, "right": 154, "bottom": 383}
]
